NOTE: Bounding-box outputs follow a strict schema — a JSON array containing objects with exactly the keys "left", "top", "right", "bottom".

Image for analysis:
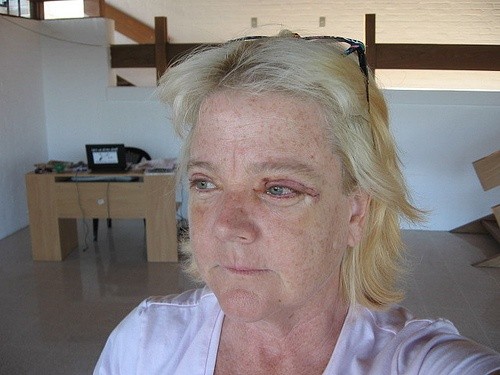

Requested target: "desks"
[{"left": 25, "top": 164, "right": 185, "bottom": 263}]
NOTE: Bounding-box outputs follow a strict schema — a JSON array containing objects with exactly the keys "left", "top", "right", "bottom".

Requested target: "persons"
[{"left": 92, "top": 31, "right": 498, "bottom": 374}]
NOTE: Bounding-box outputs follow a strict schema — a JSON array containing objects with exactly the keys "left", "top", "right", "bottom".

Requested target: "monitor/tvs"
[{"left": 85, "top": 144, "right": 126, "bottom": 171}]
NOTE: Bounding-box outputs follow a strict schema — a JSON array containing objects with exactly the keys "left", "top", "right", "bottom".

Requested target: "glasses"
[{"left": 222, "top": 35, "right": 371, "bottom": 115}]
